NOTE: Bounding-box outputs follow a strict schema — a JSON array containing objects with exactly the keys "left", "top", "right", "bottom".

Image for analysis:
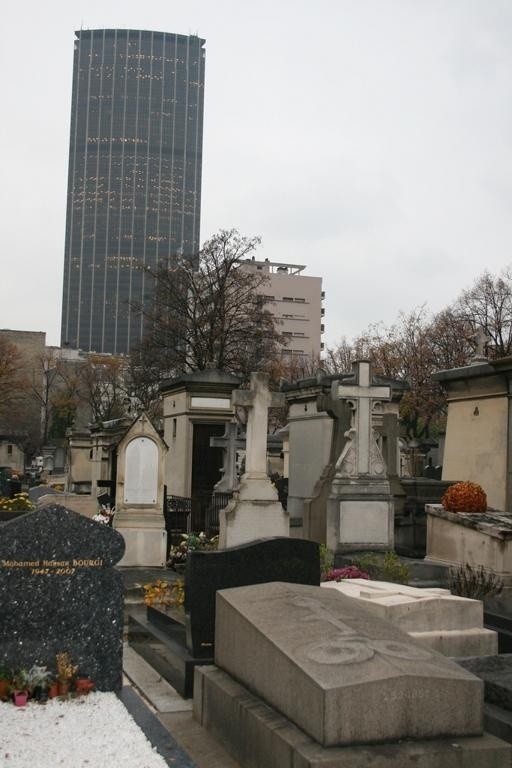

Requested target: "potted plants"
[{"left": 0, "top": 654, "right": 100, "bottom": 706}]
